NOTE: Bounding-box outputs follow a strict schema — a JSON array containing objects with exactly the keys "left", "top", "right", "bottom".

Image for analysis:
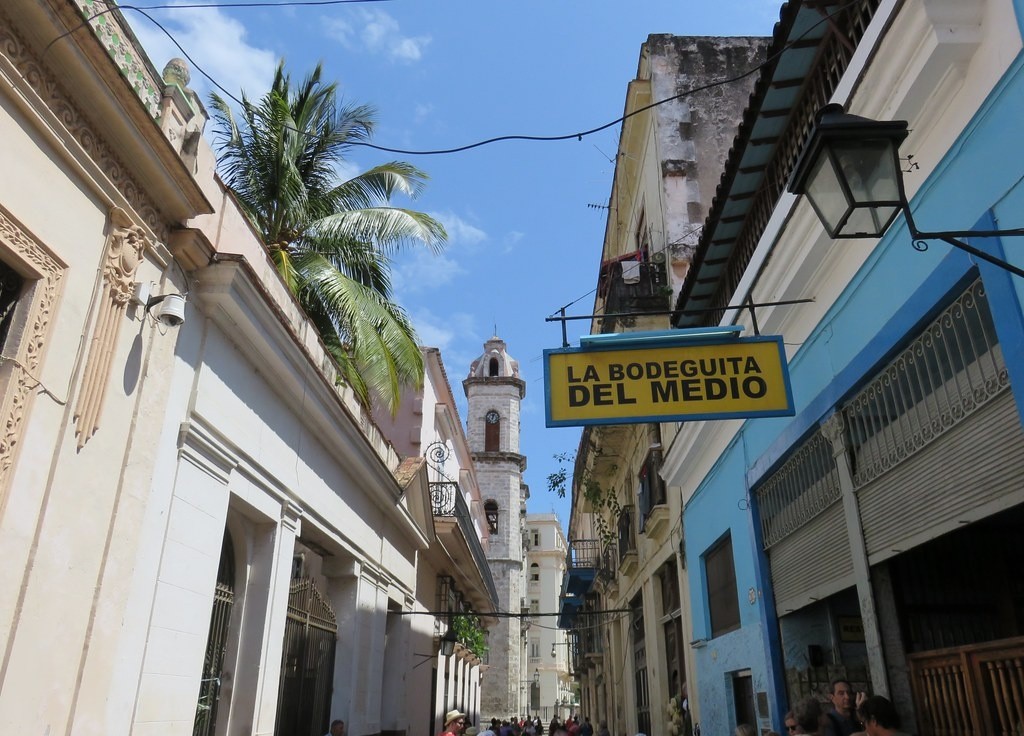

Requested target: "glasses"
[
  {"left": 785, "top": 723, "right": 799, "bottom": 732},
  {"left": 453, "top": 720, "right": 465, "bottom": 725}
]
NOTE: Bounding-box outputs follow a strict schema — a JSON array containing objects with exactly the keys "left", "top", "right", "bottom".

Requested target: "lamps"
[
  {"left": 786, "top": 99, "right": 1024, "bottom": 275},
  {"left": 140, "top": 289, "right": 186, "bottom": 334},
  {"left": 432, "top": 627, "right": 459, "bottom": 657}
]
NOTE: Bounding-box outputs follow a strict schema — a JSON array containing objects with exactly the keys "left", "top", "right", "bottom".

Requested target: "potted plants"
[{"left": 454, "top": 608, "right": 487, "bottom": 666}]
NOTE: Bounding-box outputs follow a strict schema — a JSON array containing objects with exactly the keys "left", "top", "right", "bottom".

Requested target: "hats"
[{"left": 444, "top": 710, "right": 466, "bottom": 726}]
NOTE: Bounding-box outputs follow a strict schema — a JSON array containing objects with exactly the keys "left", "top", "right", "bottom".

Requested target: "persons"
[
  {"left": 735, "top": 676, "right": 916, "bottom": 736},
  {"left": 435, "top": 709, "right": 615, "bottom": 736},
  {"left": 321, "top": 719, "right": 351, "bottom": 736}
]
